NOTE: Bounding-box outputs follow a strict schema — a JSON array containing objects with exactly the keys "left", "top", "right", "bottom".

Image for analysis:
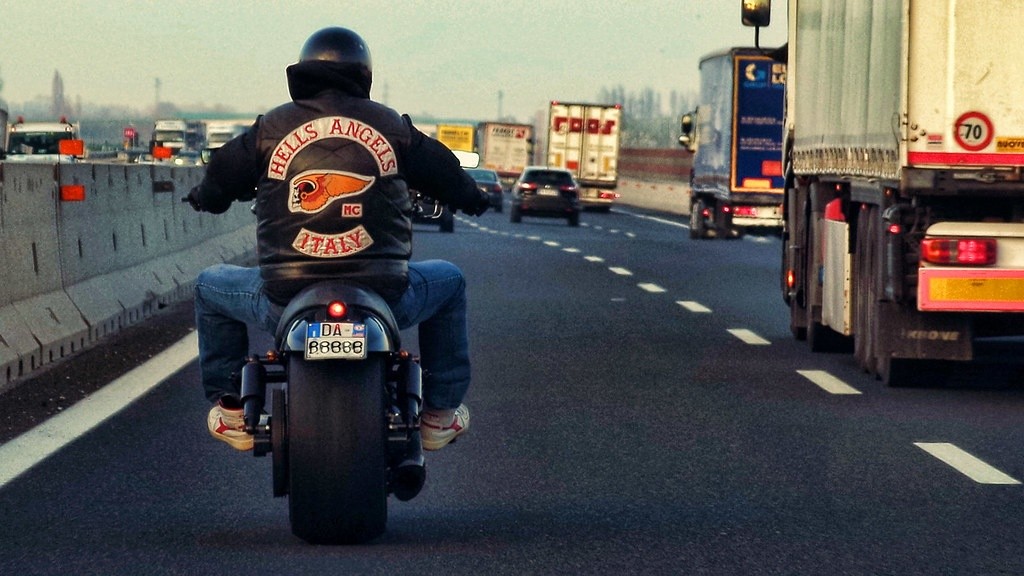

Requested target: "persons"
[{"left": 193, "top": 26, "right": 492, "bottom": 450}]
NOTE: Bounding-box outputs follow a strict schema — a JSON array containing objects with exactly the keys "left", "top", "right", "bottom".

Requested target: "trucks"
[
  {"left": 545, "top": 100, "right": 623, "bottom": 213},
  {"left": 678, "top": 43, "right": 788, "bottom": 241},
  {"left": 148, "top": 117, "right": 259, "bottom": 167},
  {"left": 4, "top": 119, "right": 89, "bottom": 164},
  {"left": 473, "top": 121, "right": 535, "bottom": 192},
  {"left": 434, "top": 122, "right": 476, "bottom": 154}
]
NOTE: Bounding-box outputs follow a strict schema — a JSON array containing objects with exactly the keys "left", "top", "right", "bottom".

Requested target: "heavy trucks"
[{"left": 741, "top": 0, "right": 1024, "bottom": 390}]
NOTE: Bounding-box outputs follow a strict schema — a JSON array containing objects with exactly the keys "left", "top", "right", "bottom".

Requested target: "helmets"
[{"left": 300, "top": 27, "right": 372, "bottom": 72}]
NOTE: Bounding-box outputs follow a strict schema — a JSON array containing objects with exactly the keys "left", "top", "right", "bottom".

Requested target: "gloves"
[
  {"left": 189, "top": 184, "right": 204, "bottom": 211},
  {"left": 461, "top": 189, "right": 492, "bottom": 219}
]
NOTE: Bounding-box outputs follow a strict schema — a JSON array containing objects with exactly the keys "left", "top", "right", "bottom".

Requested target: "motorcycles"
[{"left": 181, "top": 144, "right": 480, "bottom": 545}]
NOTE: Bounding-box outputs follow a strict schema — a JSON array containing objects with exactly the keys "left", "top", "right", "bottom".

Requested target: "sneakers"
[
  {"left": 207, "top": 399, "right": 255, "bottom": 451},
  {"left": 420, "top": 399, "right": 469, "bottom": 452}
]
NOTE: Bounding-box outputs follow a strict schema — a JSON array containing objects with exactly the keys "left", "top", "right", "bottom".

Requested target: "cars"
[
  {"left": 507, "top": 165, "right": 584, "bottom": 227},
  {"left": 460, "top": 167, "right": 505, "bottom": 214}
]
